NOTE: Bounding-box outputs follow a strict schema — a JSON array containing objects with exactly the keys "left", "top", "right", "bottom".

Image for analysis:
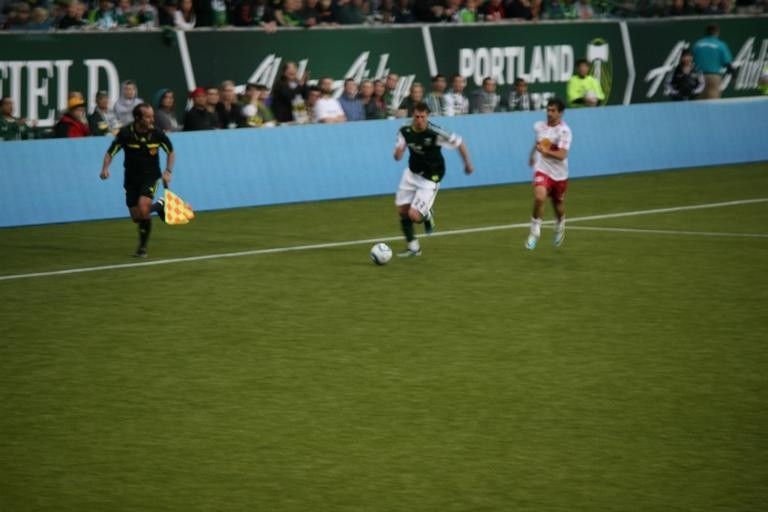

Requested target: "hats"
[
  {"left": 191, "top": 87, "right": 207, "bottom": 97},
  {"left": 66, "top": 98, "right": 85, "bottom": 109}
]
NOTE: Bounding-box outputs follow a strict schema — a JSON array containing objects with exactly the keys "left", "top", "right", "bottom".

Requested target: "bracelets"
[{"left": 165, "top": 167, "right": 172, "bottom": 175}]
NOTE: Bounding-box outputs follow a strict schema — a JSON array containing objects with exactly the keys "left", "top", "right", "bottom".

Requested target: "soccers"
[{"left": 369, "top": 243, "right": 392, "bottom": 264}]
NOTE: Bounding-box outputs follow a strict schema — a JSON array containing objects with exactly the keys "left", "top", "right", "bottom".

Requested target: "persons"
[
  {"left": 566, "top": 58, "right": 605, "bottom": 108},
  {"left": 663, "top": 50, "right": 706, "bottom": 100},
  {"left": 754, "top": 59, "right": 768, "bottom": 95},
  {"left": 391, "top": 103, "right": 473, "bottom": 258},
  {"left": 691, "top": 25, "right": 738, "bottom": 100},
  {"left": 271, "top": 61, "right": 309, "bottom": 123},
  {"left": 525, "top": 98, "right": 572, "bottom": 249},
  {"left": 100, "top": 103, "right": 176, "bottom": 258}
]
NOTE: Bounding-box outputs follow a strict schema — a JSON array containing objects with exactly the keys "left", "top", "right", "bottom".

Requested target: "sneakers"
[
  {"left": 156, "top": 197, "right": 168, "bottom": 223},
  {"left": 552, "top": 220, "right": 567, "bottom": 247},
  {"left": 133, "top": 247, "right": 148, "bottom": 259},
  {"left": 524, "top": 229, "right": 541, "bottom": 251},
  {"left": 396, "top": 246, "right": 423, "bottom": 259},
  {"left": 424, "top": 207, "right": 436, "bottom": 235}
]
